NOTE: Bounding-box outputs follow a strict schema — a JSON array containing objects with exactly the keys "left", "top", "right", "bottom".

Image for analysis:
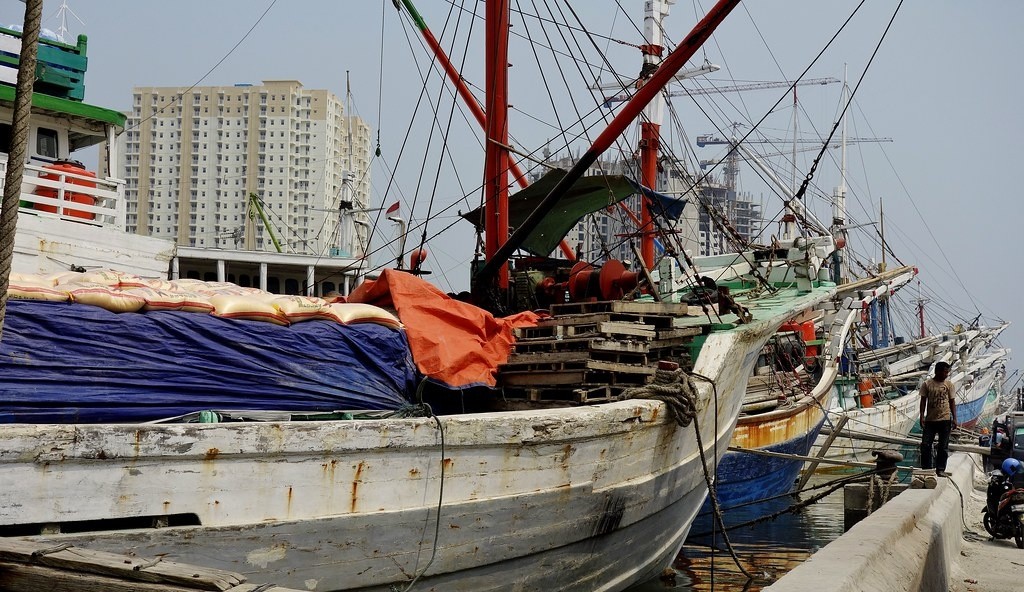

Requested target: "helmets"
[{"left": 1002, "top": 457, "right": 1020, "bottom": 476}]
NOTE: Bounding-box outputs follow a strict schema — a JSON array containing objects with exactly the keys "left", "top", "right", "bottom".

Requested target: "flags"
[{"left": 386, "top": 201, "right": 400, "bottom": 220}]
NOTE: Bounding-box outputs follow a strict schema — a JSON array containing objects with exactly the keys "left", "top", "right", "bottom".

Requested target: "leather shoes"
[{"left": 937, "top": 469, "right": 951, "bottom": 477}]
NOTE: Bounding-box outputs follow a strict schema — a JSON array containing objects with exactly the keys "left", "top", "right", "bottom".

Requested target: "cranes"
[
  {"left": 604, "top": 76, "right": 841, "bottom": 108},
  {"left": 699, "top": 142, "right": 857, "bottom": 169},
  {"left": 697, "top": 122, "right": 894, "bottom": 147}
]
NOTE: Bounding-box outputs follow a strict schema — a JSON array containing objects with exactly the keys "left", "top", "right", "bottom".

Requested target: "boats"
[
  {"left": 637, "top": 2, "right": 1010, "bottom": 512},
  {"left": 0, "top": 0, "right": 836, "bottom": 592}
]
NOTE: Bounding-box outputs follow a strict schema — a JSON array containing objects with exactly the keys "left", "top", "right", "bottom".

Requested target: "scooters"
[{"left": 981, "top": 470, "right": 1024, "bottom": 548}]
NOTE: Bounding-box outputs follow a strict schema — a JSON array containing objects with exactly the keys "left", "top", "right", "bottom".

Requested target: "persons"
[
  {"left": 979, "top": 425, "right": 1009, "bottom": 474},
  {"left": 918, "top": 362, "right": 958, "bottom": 477}
]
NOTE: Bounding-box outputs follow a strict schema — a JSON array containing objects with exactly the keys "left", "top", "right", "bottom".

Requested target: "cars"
[{"left": 991, "top": 412, "right": 1024, "bottom": 465}]
